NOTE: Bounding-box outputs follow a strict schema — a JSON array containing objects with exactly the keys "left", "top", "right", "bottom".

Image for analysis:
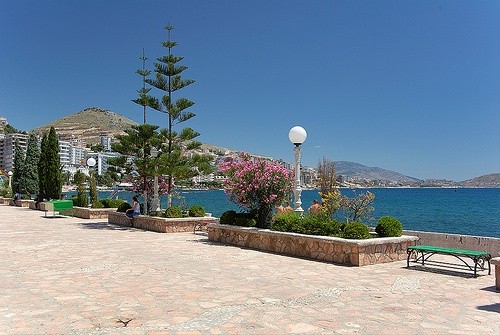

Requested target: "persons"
[
  {"left": 275, "top": 202, "right": 284, "bottom": 216},
  {"left": 284, "top": 201, "right": 293, "bottom": 216},
  {"left": 34, "top": 189, "right": 46, "bottom": 210},
  {"left": 12, "top": 190, "right": 20, "bottom": 207},
  {"left": 125, "top": 196, "right": 141, "bottom": 227},
  {"left": 307, "top": 199, "right": 322, "bottom": 216}
]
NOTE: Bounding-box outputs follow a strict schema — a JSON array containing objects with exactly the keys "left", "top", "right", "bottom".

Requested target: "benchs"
[{"left": 406, "top": 245, "right": 492, "bottom": 278}]
